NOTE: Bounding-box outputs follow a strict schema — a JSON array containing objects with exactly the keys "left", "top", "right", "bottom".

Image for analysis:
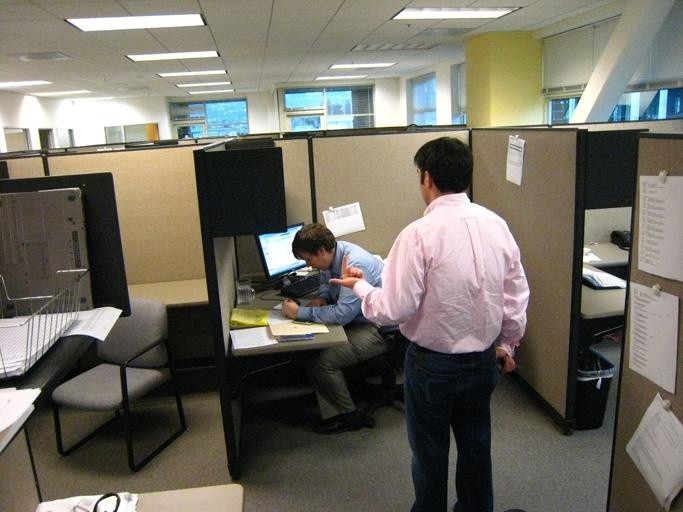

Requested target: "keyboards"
[{"left": 281, "top": 273, "right": 320, "bottom": 298}]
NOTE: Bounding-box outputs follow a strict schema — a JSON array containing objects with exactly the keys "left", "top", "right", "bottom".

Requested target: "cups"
[{"left": 236, "top": 279, "right": 254, "bottom": 307}]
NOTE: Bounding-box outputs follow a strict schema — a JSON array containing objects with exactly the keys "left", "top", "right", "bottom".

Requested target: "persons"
[
  {"left": 325, "top": 135, "right": 530, "bottom": 510},
  {"left": 281, "top": 222, "right": 386, "bottom": 435}
]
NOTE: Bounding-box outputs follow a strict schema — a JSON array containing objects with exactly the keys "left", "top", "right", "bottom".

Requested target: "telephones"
[{"left": 611, "top": 231, "right": 632, "bottom": 247}]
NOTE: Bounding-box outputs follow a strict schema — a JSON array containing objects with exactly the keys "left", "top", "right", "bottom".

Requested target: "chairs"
[
  {"left": 50, "top": 297, "right": 188, "bottom": 473},
  {"left": 339, "top": 325, "right": 405, "bottom": 423}
]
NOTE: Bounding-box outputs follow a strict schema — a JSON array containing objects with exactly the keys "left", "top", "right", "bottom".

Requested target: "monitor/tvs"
[{"left": 254, "top": 222, "right": 305, "bottom": 289}]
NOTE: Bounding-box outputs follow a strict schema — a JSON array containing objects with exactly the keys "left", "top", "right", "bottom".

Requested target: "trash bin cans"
[{"left": 574, "top": 348, "right": 616, "bottom": 430}]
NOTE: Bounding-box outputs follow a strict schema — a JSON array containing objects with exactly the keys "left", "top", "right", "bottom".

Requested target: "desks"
[
  {"left": 36, "top": 483, "right": 245, "bottom": 511},
  {"left": 0, "top": 310, "right": 97, "bottom": 512},
  {"left": 229, "top": 273, "right": 349, "bottom": 357},
  {"left": 579, "top": 241, "right": 631, "bottom": 320}
]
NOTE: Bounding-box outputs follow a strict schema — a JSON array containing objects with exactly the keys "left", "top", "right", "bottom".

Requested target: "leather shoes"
[{"left": 317, "top": 417, "right": 362, "bottom": 434}]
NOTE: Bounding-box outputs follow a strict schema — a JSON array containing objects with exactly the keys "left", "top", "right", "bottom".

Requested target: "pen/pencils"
[{"left": 292, "top": 321, "right": 312, "bottom": 325}]
